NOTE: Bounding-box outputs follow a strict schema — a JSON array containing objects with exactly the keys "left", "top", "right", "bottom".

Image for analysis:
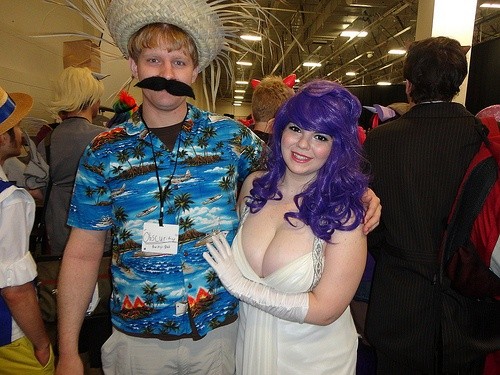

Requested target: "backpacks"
[{"left": 436, "top": 116, "right": 499, "bottom": 298}]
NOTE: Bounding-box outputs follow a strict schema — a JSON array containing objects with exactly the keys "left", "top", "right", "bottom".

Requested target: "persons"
[
  {"left": 0, "top": 93, "right": 56, "bottom": 375},
  {"left": 107, "top": 95, "right": 139, "bottom": 131},
  {"left": 54, "top": 16, "right": 382, "bottom": 374},
  {"left": 27, "top": 65, "right": 113, "bottom": 367},
  {"left": 202, "top": 79, "right": 367, "bottom": 375},
  {"left": 362, "top": 36, "right": 500, "bottom": 375},
  {"left": 251, "top": 75, "right": 295, "bottom": 148}
]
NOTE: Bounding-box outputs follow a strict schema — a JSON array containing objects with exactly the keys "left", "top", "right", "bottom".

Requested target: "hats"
[
  {"left": 106, "top": 0, "right": 225, "bottom": 73},
  {"left": 0, "top": 88, "right": 34, "bottom": 134}
]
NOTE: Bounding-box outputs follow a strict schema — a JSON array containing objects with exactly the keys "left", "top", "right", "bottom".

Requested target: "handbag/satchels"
[{"left": 33, "top": 251, "right": 111, "bottom": 321}]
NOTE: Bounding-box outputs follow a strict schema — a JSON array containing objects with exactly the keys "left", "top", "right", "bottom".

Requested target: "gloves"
[{"left": 202, "top": 235, "right": 309, "bottom": 325}]
